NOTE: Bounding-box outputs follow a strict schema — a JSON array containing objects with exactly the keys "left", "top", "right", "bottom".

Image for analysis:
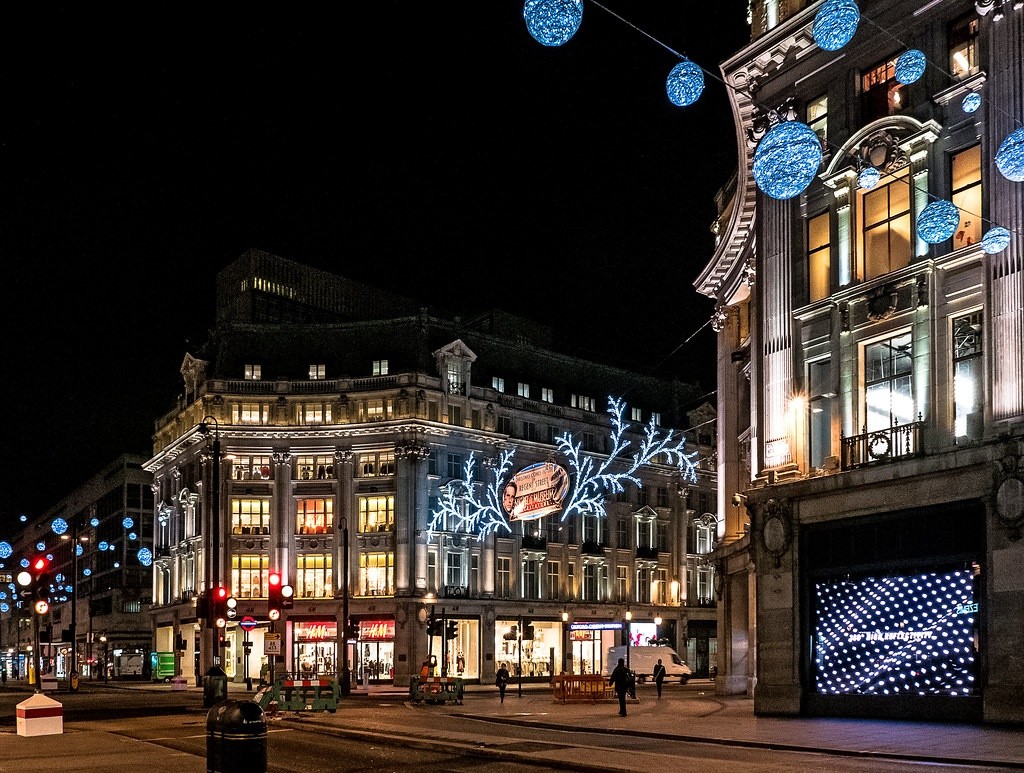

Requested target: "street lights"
[
  {"left": 61, "top": 529, "right": 92, "bottom": 692},
  {"left": 625, "top": 610, "right": 633, "bottom": 670},
  {"left": 655, "top": 616, "right": 663, "bottom": 646},
  {"left": 562, "top": 612, "right": 569, "bottom": 704}
]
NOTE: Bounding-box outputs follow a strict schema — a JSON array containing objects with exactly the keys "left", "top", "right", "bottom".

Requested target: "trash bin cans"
[
  {"left": 203, "top": 665, "right": 228, "bottom": 709},
  {"left": 206, "top": 700, "right": 267, "bottom": 773}
]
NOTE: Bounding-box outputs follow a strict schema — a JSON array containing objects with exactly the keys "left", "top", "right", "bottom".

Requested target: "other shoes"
[
  {"left": 618, "top": 711, "right": 627, "bottom": 717},
  {"left": 658, "top": 694, "right": 661, "bottom": 700},
  {"left": 500, "top": 699, "right": 504, "bottom": 703}
]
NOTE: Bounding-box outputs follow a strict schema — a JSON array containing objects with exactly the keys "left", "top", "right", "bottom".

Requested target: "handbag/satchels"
[
  {"left": 495, "top": 676, "right": 503, "bottom": 687},
  {"left": 652, "top": 677, "right": 657, "bottom": 681}
]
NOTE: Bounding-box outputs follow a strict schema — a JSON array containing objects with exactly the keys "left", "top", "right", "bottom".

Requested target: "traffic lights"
[
  {"left": 449, "top": 621, "right": 459, "bottom": 640},
  {"left": 214, "top": 587, "right": 238, "bottom": 628},
  {"left": 267, "top": 573, "right": 294, "bottom": 622},
  {"left": 18, "top": 558, "right": 50, "bottom": 616}
]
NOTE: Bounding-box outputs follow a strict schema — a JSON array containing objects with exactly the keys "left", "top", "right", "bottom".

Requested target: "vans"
[{"left": 606, "top": 646, "right": 692, "bottom": 684}]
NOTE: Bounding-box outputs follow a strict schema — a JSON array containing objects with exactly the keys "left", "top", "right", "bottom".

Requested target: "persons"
[
  {"left": 610, "top": 658, "right": 631, "bottom": 718},
  {"left": 420, "top": 655, "right": 437, "bottom": 678},
  {"left": 653, "top": 659, "right": 666, "bottom": 699},
  {"left": 325, "top": 653, "right": 332, "bottom": 673},
  {"left": 495, "top": 663, "right": 509, "bottom": 703},
  {"left": 457, "top": 651, "right": 466, "bottom": 673},
  {"left": 503, "top": 482, "right": 517, "bottom": 513}
]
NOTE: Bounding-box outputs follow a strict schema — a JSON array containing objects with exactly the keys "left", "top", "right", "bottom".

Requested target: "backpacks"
[{"left": 421, "top": 666, "right": 429, "bottom": 677}]
